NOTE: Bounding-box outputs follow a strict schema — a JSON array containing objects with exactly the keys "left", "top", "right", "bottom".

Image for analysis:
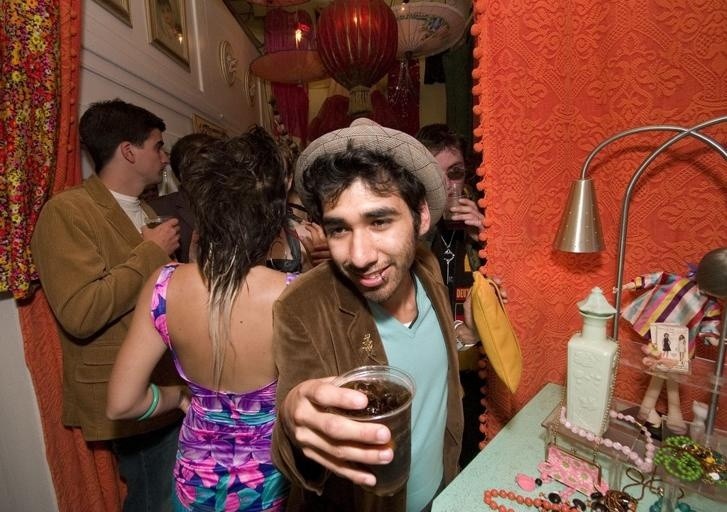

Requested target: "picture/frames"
[{"left": 145, "top": 0, "right": 191, "bottom": 75}]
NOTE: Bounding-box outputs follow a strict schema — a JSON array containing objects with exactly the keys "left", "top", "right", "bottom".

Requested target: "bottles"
[{"left": 567, "top": 285, "right": 620, "bottom": 435}]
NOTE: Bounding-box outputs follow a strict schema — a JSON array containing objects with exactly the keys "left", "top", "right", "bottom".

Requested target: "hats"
[{"left": 294, "top": 118, "right": 447, "bottom": 230}]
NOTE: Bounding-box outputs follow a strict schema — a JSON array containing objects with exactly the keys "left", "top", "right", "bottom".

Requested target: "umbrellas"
[
  {"left": 387, "top": 1, "right": 465, "bottom": 65},
  {"left": 249, "top": 37, "right": 330, "bottom": 88}
]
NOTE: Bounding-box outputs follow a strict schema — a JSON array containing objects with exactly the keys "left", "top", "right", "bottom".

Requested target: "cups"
[
  {"left": 144, "top": 215, "right": 173, "bottom": 229},
  {"left": 442, "top": 182, "right": 473, "bottom": 228},
  {"left": 329, "top": 365, "right": 417, "bottom": 496}
]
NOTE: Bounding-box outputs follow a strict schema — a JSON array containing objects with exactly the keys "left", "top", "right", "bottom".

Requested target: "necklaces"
[
  {"left": 559, "top": 405, "right": 654, "bottom": 473},
  {"left": 654, "top": 433, "right": 726, "bottom": 488},
  {"left": 482, "top": 467, "right": 694, "bottom": 511}
]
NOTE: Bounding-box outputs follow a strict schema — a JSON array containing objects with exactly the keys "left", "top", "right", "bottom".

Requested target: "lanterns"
[{"left": 315, "top": 0, "right": 397, "bottom": 118}]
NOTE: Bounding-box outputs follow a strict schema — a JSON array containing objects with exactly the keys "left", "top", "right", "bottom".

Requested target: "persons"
[
  {"left": 612, "top": 263, "right": 719, "bottom": 434},
  {"left": 674, "top": 335, "right": 686, "bottom": 363},
  {"left": 33, "top": 98, "right": 508, "bottom": 511},
  {"left": 660, "top": 332, "right": 671, "bottom": 358}
]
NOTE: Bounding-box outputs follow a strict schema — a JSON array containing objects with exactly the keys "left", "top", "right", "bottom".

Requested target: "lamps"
[{"left": 249, "top": 0, "right": 470, "bottom": 114}]
